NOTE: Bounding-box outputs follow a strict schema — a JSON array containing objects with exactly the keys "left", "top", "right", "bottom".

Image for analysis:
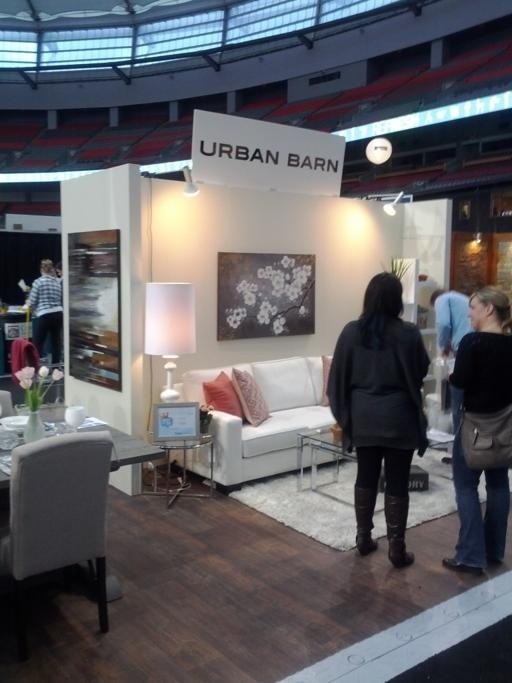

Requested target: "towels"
[{"left": 10, "top": 337, "right": 59, "bottom": 382}]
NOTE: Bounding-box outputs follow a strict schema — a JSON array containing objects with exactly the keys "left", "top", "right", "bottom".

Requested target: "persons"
[
  {"left": 327, "top": 272, "right": 431, "bottom": 567},
  {"left": 442, "top": 286, "right": 511, "bottom": 577},
  {"left": 430, "top": 289, "right": 475, "bottom": 465},
  {"left": 23, "top": 260, "right": 63, "bottom": 372},
  {"left": 56, "top": 262, "right": 62, "bottom": 278}
]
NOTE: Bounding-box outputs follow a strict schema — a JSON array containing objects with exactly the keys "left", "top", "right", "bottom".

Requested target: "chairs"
[
  {"left": 1, "top": 429, "right": 114, "bottom": 662},
  {"left": 0, "top": 390, "right": 14, "bottom": 419}
]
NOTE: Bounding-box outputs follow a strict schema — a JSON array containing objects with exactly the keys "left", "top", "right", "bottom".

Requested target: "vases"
[
  {"left": 201, "top": 423, "right": 210, "bottom": 434},
  {"left": 23, "top": 411, "right": 44, "bottom": 445}
]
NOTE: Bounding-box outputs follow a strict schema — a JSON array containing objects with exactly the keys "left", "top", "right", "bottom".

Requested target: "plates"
[{"left": 0, "top": 415, "right": 29, "bottom": 428}]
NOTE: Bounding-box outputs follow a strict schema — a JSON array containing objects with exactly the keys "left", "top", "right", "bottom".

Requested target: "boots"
[
  {"left": 384, "top": 492, "right": 415, "bottom": 568},
  {"left": 355, "top": 484, "right": 378, "bottom": 556}
]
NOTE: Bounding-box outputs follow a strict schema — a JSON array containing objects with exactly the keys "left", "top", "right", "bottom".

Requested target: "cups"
[
  {"left": 65, "top": 406, "right": 85, "bottom": 432},
  {"left": 1, "top": 429, "right": 19, "bottom": 450}
]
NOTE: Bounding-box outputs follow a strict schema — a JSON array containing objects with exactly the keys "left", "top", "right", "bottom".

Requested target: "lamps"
[
  {"left": 146, "top": 281, "right": 195, "bottom": 402},
  {"left": 180, "top": 165, "right": 199, "bottom": 197},
  {"left": 383, "top": 191, "right": 404, "bottom": 216}
]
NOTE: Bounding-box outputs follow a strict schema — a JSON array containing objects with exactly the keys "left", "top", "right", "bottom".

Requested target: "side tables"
[{"left": 141, "top": 430, "right": 215, "bottom": 507}]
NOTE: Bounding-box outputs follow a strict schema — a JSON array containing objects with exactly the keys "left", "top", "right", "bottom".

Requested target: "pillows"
[
  {"left": 322, "top": 356, "right": 332, "bottom": 406},
  {"left": 202, "top": 371, "right": 247, "bottom": 421},
  {"left": 230, "top": 367, "right": 272, "bottom": 426}
]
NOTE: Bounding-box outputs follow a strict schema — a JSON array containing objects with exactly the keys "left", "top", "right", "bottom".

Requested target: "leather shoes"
[{"left": 442, "top": 557, "right": 484, "bottom": 574}]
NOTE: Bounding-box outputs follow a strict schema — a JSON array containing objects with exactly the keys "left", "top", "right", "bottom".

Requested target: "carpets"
[{"left": 228, "top": 445, "right": 512, "bottom": 552}]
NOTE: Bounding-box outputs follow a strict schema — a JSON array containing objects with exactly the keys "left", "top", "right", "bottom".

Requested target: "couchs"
[{"left": 164, "top": 356, "right": 357, "bottom": 496}]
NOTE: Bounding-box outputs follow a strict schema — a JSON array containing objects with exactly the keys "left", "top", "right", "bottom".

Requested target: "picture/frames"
[{"left": 153, "top": 403, "right": 200, "bottom": 442}]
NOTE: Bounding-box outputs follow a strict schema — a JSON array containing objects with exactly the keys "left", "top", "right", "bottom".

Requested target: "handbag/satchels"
[{"left": 461, "top": 408, "right": 511, "bottom": 470}]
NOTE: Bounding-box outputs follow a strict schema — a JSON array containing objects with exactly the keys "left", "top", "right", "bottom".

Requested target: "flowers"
[
  {"left": 198, "top": 402, "right": 214, "bottom": 425},
  {"left": 224, "top": 255, "right": 315, "bottom": 336},
  {"left": 13, "top": 365, "right": 64, "bottom": 428}
]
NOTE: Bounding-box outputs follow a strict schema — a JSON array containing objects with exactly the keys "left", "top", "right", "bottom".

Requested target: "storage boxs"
[{"left": 408, "top": 464, "right": 429, "bottom": 490}]
